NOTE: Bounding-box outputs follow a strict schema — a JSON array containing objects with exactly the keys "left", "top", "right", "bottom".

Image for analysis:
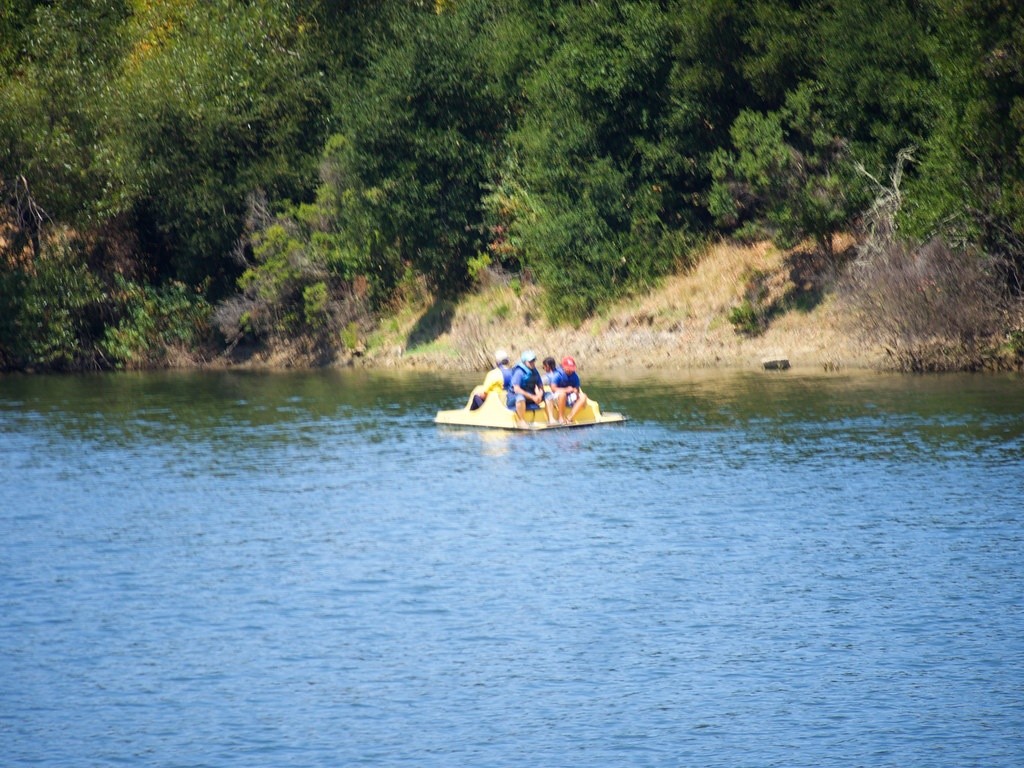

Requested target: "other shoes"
[
  {"left": 519, "top": 420, "right": 530, "bottom": 429},
  {"left": 564, "top": 415, "right": 573, "bottom": 424}
]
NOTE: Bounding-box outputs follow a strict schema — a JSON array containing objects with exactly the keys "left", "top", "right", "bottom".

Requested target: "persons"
[
  {"left": 541, "top": 357, "right": 557, "bottom": 385},
  {"left": 507, "top": 352, "right": 561, "bottom": 430},
  {"left": 465, "top": 349, "right": 511, "bottom": 410},
  {"left": 551, "top": 356, "right": 587, "bottom": 425}
]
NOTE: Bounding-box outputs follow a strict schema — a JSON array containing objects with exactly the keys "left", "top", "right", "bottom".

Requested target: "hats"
[
  {"left": 496, "top": 350, "right": 508, "bottom": 360},
  {"left": 561, "top": 356, "right": 577, "bottom": 372},
  {"left": 521, "top": 351, "right": 536, "bottom": 362}
]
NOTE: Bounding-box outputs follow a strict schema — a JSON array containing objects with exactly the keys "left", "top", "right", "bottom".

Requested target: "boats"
[{"left": 433, "top": 388, "right": 627, "bottom": 430}]
